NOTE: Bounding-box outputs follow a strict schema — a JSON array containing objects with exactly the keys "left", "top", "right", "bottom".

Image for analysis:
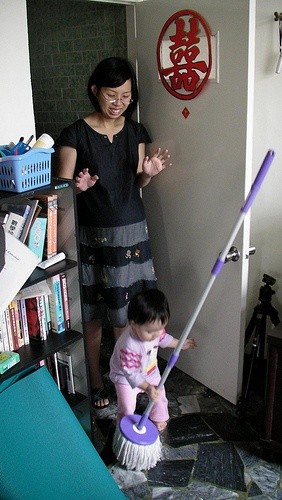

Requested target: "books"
[
  {"left": 0, "top": 272, "right": 75, "bottom": 395},
  {"left": 0, "top": 192, "right": 58, "bottom": 265}
]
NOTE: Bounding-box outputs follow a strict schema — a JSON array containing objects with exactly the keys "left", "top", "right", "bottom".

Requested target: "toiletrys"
[{"left": 37, "top": 252, "right": 67, "bottom": 269}]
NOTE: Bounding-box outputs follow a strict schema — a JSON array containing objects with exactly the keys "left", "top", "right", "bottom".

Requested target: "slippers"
[{"left": 90, "top": 387, "right": 110, "bottom": 409}]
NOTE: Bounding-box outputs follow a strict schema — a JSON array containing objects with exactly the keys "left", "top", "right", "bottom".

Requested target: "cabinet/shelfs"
[{"left": 0, "top": 178, "right": 92, "bottom": 449}]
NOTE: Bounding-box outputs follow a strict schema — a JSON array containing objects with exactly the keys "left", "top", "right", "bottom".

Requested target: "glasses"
[{"left": 98, "top": 89, "right": 134, "bottom": 104}]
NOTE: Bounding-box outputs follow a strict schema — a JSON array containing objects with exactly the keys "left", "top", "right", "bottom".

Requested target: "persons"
[
  {"left": 108, "top": 289, "right": 197, "bottom": 431},
  {"left": 56, "top": 57, "right": 172, "bottom": 410}
]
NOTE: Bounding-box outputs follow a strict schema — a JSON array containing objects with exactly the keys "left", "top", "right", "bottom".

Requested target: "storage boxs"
[{"left": 0, "top": 350, "right": 20, "bottom": 374}]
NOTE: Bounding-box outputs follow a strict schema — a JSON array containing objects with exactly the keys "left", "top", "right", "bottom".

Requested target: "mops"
[{"left": 111, "top": 148, "right": 276, "bottom": 472}]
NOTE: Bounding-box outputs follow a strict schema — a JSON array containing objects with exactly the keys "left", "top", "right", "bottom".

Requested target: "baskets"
[{"left": 0, "top": 148, "right": 55, "bottom": 193}]
